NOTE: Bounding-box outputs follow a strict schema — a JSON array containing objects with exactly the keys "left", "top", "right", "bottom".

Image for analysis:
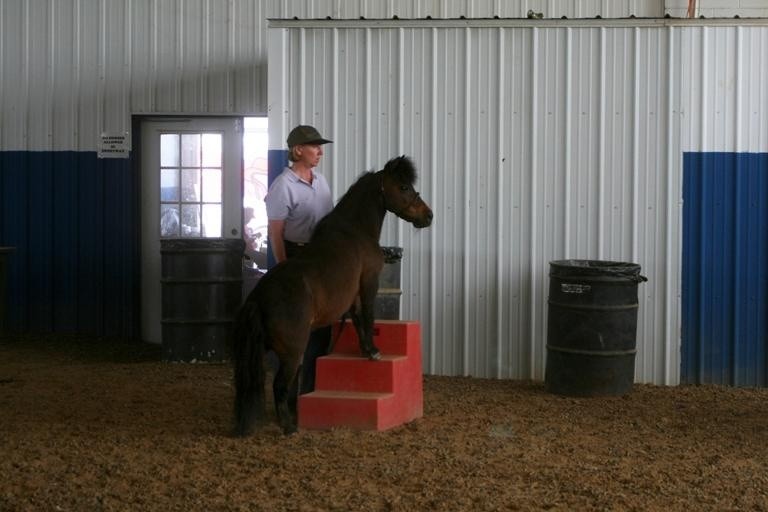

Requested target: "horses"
[{"left": 234, "top": 155, "right": 432, "bottom": 438}]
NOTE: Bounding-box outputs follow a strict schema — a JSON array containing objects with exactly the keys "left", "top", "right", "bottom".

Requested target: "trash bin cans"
[
  {"left": 159, "top": 240, "right": 244, "bottom": 361},
  {"left": 545, "top": 259, "right": 640, "bottom": 397}
]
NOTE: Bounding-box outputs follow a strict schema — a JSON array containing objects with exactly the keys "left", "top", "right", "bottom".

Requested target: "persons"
[
  {"left": 241, "top": 208, "right": 262, "bottom": 252},
  {"left": 263, "top": 125, "right": 337, "bottom": 419}
]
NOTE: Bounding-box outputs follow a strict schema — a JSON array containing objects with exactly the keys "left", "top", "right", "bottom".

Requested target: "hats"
[{"left": 286, "top": 125, "right": 334, "bottom": 148}]
